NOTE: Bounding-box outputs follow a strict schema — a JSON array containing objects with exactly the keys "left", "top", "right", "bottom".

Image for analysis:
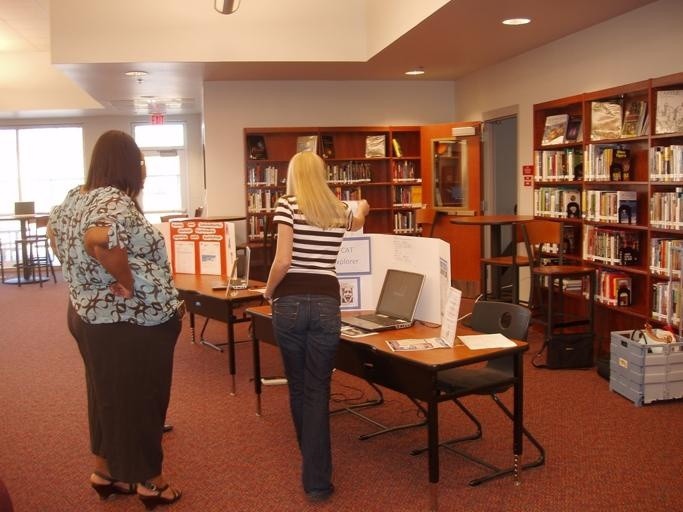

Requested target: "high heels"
[
  {"left": 135, "top": 480, "right": 182, "bottom": 510},
  {"left": 87, "top": 471, "right": 136, "bottom": 502}
]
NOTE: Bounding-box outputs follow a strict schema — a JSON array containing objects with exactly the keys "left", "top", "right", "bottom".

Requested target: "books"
[
  {"left": 321, "top": 134, "right": 387, "bottom": 201},
  {"left": 247, "top": 134, "right": 287, "bottom": 243},
  {"left": 531, "top": 90, "right": 683, "bottom": 334},
  {"left": 392, "top": 138, "right": 422, "bottom": 234}
]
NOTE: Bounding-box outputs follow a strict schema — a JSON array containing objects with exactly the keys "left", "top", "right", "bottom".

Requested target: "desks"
[
  {"left": 245, "top": 303, "right": 529, "bottom": 486},
  {"left": 450, "top": 214, "right": 534, "bottom": 300},
  {"left": 0, "top": 213, "right": 49, "bottom": 285},
  {"left": 173, "top": 274, "right": 266, "bottom": 397}
]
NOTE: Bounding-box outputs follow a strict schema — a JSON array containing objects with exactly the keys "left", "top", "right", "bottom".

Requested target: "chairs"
[
  {"left": 408, "top": 299, "right": 545, "bottom": 486},
  {"left": 480, "top": 219, "right": 543, "bottom": 303},
  {"left": 15, "top": 216, "right": 57, "bottom": 287},
  {"left": 199, "top": 255, "right": 274, "bottom": 352},
  {"left": 521, "top": 219, "right": 596, "bottom": 337},
  {"left": 160, "top": 214, "right": 189, "bottom": 223}
]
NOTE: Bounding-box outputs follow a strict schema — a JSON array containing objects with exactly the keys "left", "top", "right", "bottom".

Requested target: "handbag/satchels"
[{"left": 531, "top": 330, "right": 596, "bottom": 371}]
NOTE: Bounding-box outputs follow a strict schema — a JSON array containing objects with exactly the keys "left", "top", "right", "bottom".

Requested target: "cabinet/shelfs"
[
  {"left": 243, "top": 126, "right": 421, "bottom": 243},
  {"left": 533, "top": 71, "right": 683, "bottom": 360}
]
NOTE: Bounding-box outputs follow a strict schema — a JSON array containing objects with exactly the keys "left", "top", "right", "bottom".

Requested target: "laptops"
[
  {"left": 14, "top": 202, "right": 35, "bottom": 217},
  {"left": 211, "top": 246, "right": 251, "bottom": 289},
  {"left": 341, "top": 268, "right": 427, "bottom": 331}
]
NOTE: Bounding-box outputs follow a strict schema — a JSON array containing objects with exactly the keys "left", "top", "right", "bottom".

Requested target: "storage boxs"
[{"left": 609, "top": 327, "right": 683, "bottom": 406}]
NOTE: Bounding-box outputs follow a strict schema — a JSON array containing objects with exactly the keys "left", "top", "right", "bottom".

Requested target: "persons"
[
  {"left": 262, "top": 149, "right": 371, "bottom": 502},
  {"left": 342, "top": 287, "right": 353, "bottom": 303},
  {"left": 45, "top": 130, "right": 184, "bottom": 511}
]
